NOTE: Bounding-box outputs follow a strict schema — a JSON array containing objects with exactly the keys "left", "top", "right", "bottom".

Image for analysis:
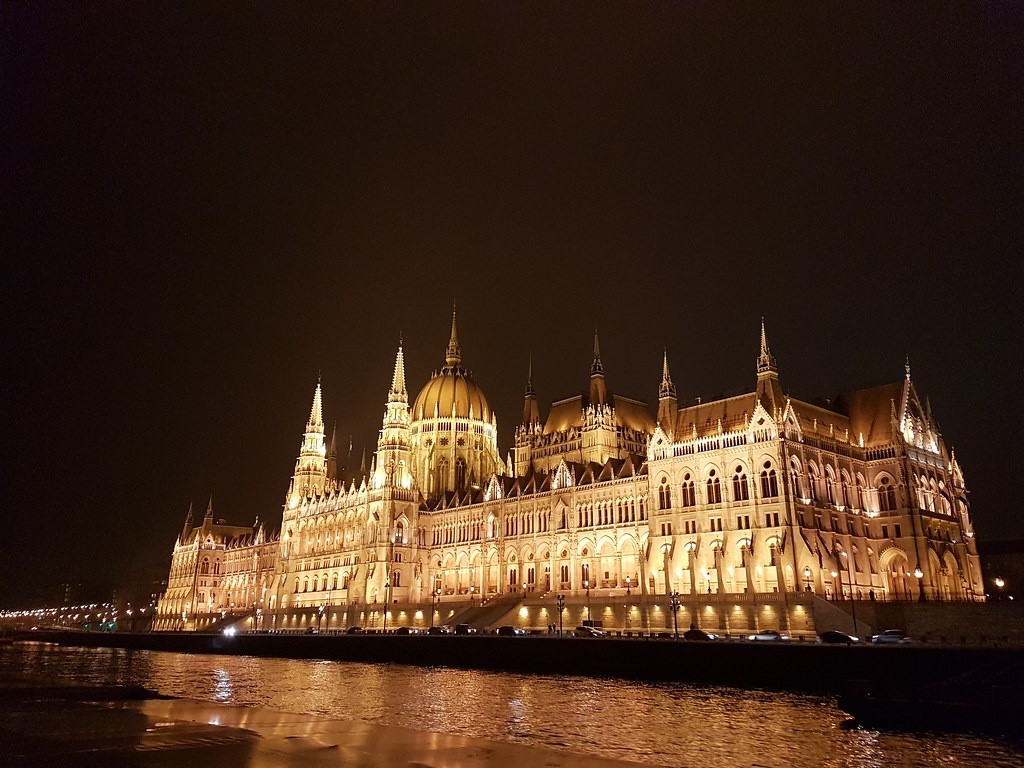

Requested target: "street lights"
[
  {"left": 914, "top": 566, "right": 926, "bottom": 602},
  {"left": 585, "top": 579, "right": 590, "bottom": 627},
  {"left": 383, "top": 583, "right": 390, "bottom": 634},
  {"left": 326, "top": 592, "right": 329, "bottom": 633},
  {"left": 831, "top": 571, "right": 838, "bottom": 600},
  {"left": 842, "top": 550, "right": 858, "bottom": 634}
]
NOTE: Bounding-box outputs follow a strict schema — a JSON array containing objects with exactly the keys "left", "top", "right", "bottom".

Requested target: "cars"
[
  {"left": 575, "top": 627, "right": 603, "bottom": 637},
  {"left": 427, "top": 626, "right": 447, "bottom": 636},
  {"left": 816, "top": 630, "right": 859, "bottom": 646},
  {"left": 684, "top": 630, "right": 718, "bottom": 641},
  {"left": 455, "top": 624, "right": 476, "bottom": 635},
  {"left": 872, "top": 630, "right": 912, "bottom": 644},
  {"left": 305, "top": 627, "right": 419, "bottom": 635},
  {"left": 749, "top": 629, "right": 789, "bottom": 642},
  {"left": 497, "top": 625, "right": 525, "bottom": 636}
]
